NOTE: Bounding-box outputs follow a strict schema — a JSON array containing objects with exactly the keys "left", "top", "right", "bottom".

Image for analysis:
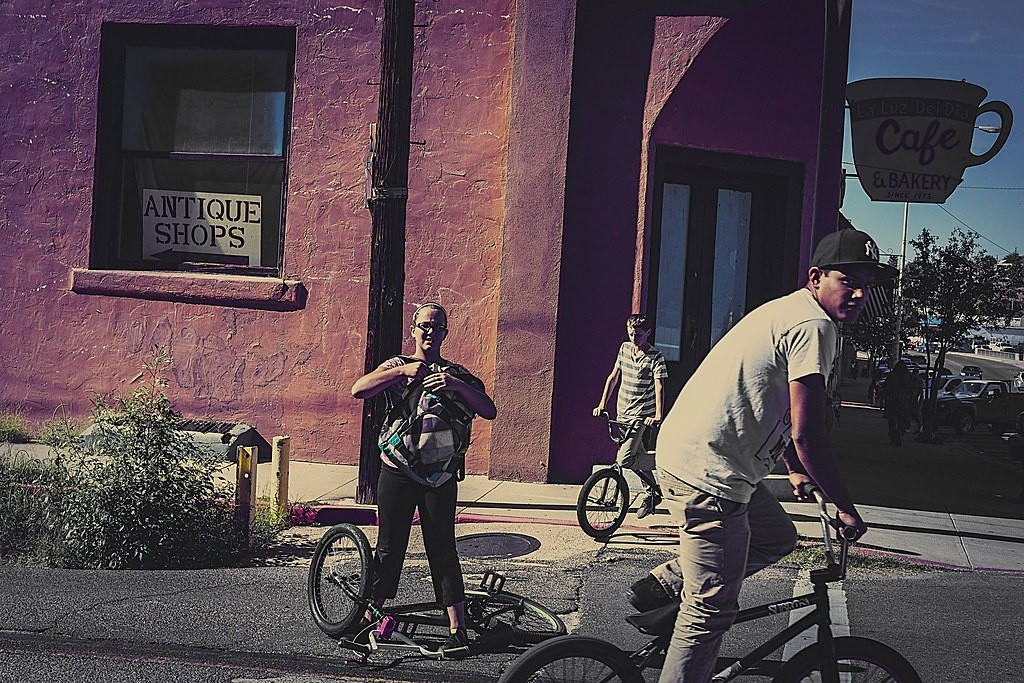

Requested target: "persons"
[
  {"left": 625, "top": 228, "right": 900, "bottom": 683},
  {"left": 343, "top": 302, "right": 497, "bottom": 659},
  {"left": 848, "top": 360, "right": 924, "bottom": 448},
  {"left": 592, "top": 314, "right": 668, "bottom": 520}
]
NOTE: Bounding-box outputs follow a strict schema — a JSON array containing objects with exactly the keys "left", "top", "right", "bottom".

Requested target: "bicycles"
[
  {"left": 496, "top": 481, "right": 923, "bottom": 683},
  {"left": 305, "top": 522, "right": 567, "bottom": 667},
  {"left": 576, "top": 409, "right": 664, "bottom": 538}
]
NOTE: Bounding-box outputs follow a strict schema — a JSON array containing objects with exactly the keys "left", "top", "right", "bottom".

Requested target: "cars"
[
  {"left": 1012, "top": 372, "right": 1024, "bottom": 388},
  {"left": 916, "top": 343, "right": 936, "bottom": 353},
  {"left": 958, "top": 364, "right": 983, "bottom": 380},
  {"left": 861, "top": 355, "right": 1024, "bottom": 434},
  {"left": 992, "top": 342, "right": 1015, "bottom": 352}
]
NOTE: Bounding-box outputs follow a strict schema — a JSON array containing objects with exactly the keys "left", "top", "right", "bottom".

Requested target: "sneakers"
[
  {"left": 636, "top": 493, "right": 662, "bottom": 519},
  {"left": 625, "top": 575, "right": 672, "bottom": 613},
  {"left": 349, "top": 618, "right": 376, "bottom": 644},
  {"left": 435, "top": 627, "right": 471, "bottom": 656}
]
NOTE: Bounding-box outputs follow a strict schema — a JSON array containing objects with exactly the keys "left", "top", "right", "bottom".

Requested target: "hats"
[{"left": 808, "top": 219, "right": 900, "bottom": 283}]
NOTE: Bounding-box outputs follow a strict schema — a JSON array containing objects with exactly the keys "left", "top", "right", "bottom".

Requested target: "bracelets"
[{"left": 837, "top": 504, "right": 853, "bottom": 513}]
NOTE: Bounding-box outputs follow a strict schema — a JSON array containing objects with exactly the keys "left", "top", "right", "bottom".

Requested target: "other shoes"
[{"left": 889, "top": 439, "right": 901, "bottom": 447}]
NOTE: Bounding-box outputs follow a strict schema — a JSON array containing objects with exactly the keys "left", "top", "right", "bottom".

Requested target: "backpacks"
[{"left": 374, "top": 353, "right": 475, "bottom": 489}]
